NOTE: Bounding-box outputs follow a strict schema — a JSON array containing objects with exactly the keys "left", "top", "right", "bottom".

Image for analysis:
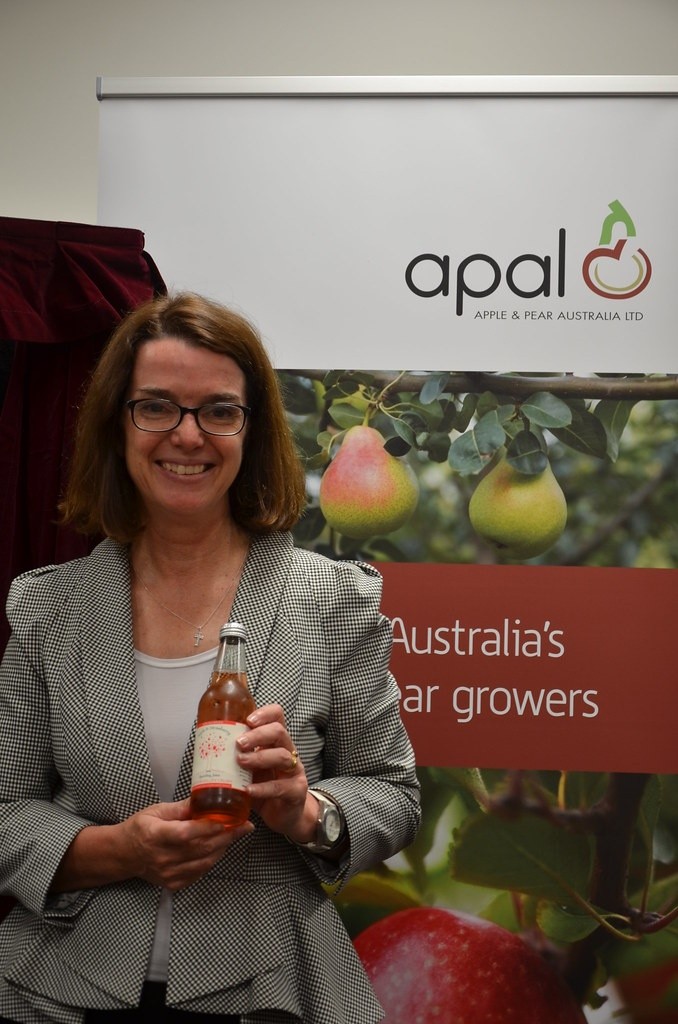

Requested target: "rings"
[{"left": 286, "top": 750, "right": 299, "bottom": 771}]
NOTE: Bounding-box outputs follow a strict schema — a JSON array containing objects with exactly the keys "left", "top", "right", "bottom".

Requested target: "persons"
[{"left": 0, "top": 294, "right": 423, "bottom": 1024}]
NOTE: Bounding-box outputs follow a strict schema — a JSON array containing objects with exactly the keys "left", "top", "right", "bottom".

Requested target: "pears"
[
  {"left": 467, "top": 452, "right": 567, "bottom": 560},
  {"left": 319, "top": 425, "right": 419, "bottom": 538}
]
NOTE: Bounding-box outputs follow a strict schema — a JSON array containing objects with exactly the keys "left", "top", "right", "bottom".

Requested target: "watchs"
[{"left": 283, "top": 790, "right": 339, "bottom": 851}]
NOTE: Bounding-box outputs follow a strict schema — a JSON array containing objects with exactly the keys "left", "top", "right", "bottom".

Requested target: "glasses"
[{"left": 123, "top": 398, "right": 252, "bottom": 437}]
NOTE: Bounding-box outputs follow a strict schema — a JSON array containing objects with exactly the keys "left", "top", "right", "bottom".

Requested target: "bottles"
[{"left": 189, "top": 622, "right": 257, "bottom": 828}]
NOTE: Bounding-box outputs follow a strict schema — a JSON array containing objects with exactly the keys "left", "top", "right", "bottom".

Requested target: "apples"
[{"left": 344, "top": 907, "right": 588, "bottom": 1024}]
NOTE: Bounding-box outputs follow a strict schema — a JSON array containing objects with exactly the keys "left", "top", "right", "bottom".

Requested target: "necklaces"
[{"left": 128, "top": 530, "right": 249, "bottom": 648}]
